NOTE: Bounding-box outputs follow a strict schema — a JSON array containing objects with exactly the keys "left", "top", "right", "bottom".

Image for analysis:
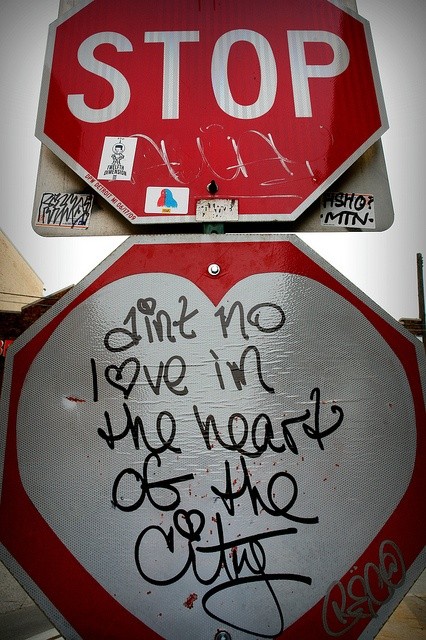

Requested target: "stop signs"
[{"left": 34, "top": 0, "right": 390, "bottom": 225}]
